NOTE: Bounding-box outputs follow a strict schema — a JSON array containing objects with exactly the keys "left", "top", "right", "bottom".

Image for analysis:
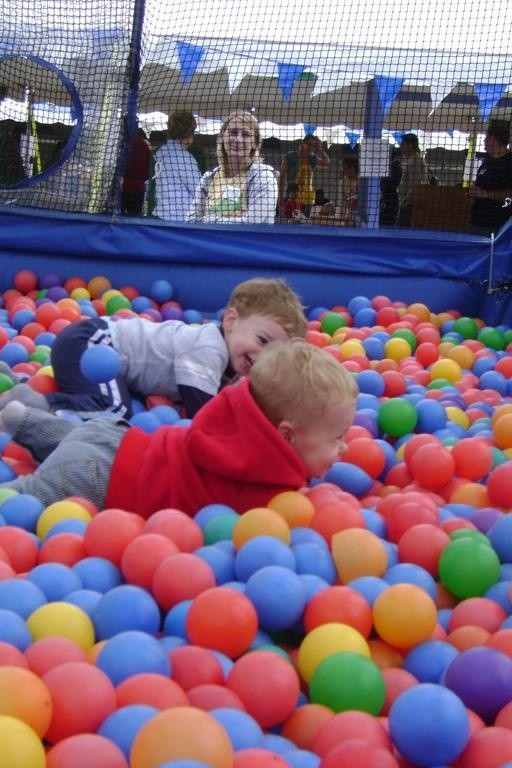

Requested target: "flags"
[{"left": 460, "top": 125, "right": 476, "bottom": 191}]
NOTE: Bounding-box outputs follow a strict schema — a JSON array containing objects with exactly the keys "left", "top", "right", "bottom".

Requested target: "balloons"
[{"left": 0, "top": 485, "right": 510, "bottom": 767}]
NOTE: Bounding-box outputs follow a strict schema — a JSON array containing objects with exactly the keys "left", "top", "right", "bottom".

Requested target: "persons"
[
  {"left": 182, "top": 109, "right": 281, "bottom": 225},
  {"left": 313, "top": 187, "right": 329, "bottom": 207},
  {"left": 396, "top": 132, "right": 435, "bottom": 227},
  {"left": 115, "top": 111, "right": 152, "bottom": 219},
  {"left": 335, "top": 157, "right": 361, "bottom": 226},
  {"left": 275, "top": 133, "right": 330, "bottom": 218},
  {"left": 378, "top": 147, "right": 404, "bottom": 226},
  {"left": 0, "top": 337, "right": 359, "bottom": 515},
  {"left": 281, "top": 183, "right": 303, "bottom": 225},
  {"left": 0, "top": 276, "right": 305, "bottom": 428},
  {"left": 466, "top": 117, "right": 511, "bottom": 237},
  {"left": 152, "top": 109, "right": 210, "bottom": 223}
]
